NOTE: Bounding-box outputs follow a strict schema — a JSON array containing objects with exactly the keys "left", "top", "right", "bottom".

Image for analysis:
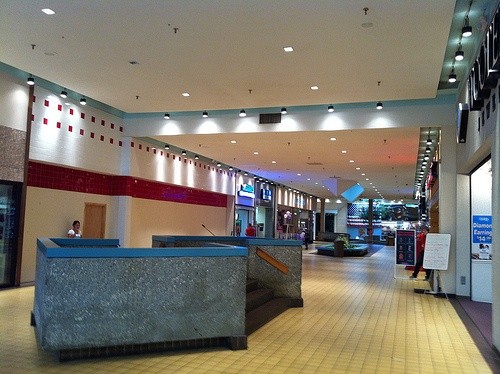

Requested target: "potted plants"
[{"left": 333, "top": 235, "right": 350, "bottom": 257}]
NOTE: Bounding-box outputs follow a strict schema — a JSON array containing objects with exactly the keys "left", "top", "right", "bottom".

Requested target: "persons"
[
  {"left": 304, "top": 230, "right": 310, "bottom": 250},
  {"left": 245, "top": 223, "right": 256, "bottom": 236},
  {"left": 388, "top": 231, "right": 394, "bottom": 236},
  {"left": 67, "top": 220, "right": 82, "bottom": 238},
  {"left": 408, "top": 226, "right": 432, "bottom": 281}
]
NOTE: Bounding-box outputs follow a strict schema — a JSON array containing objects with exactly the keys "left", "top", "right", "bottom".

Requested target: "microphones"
[{"left": 202, "top": 224, "right": 215, "bottom": 235}]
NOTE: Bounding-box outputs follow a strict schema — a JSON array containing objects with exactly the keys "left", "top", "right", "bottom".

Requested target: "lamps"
[
  {"left": 462, "top": 15, "right": 473, "bottom": 37},
  {"left": 216, "top": 162, "right": 221, "bottom": 166},
  {"left": 79, "top": 96, "right": 86, "bottom": 106},
  {"left": 182, "top": 150, "right": 187, "bottom": 155},
  {"left": 228, "top": 166, "right": 234, "bottom": 170},
  {"left": 376, "top": 101, "right": 384, "bottom": 110},
  {"left": 164, "top": 112, "right": 170, "bottom": 120},
  {"left": 281, "top": 107, "right": 287, "bottom": 115},
  {"left": 202, "top": 110, "right": 208, "bottom": 118},
  {"left": 327, "top": 104, "right": 335, "bottom": 112},
  {"left": 454, "top": 45, "right": 465, "bottom": 61},
  {"left": 60, "top": 87, "right": 69, "bottom": 99},
  {"left": 253, "top": 175, "right": 274, "bottom": 185},
  {"left": 448, "top": 69, "right": 457, "bottom": 83},
  {"left": 165, "top": 145, "right": 170, "bottom": 149},
  {"left": 27, "top": 73, "right": 35, "bottom": 85},
  {"left": 239, "top": 109, "right": 247, "bottom": 118},
  {"left": 415, "top": 136, "right": 432, "bottom": 200},
  {"left": 244, "top": 171, "right": 249, "bottom": 175},
  {"left": 194, "top": 154, "right": 199, "bottom": 159}
]
